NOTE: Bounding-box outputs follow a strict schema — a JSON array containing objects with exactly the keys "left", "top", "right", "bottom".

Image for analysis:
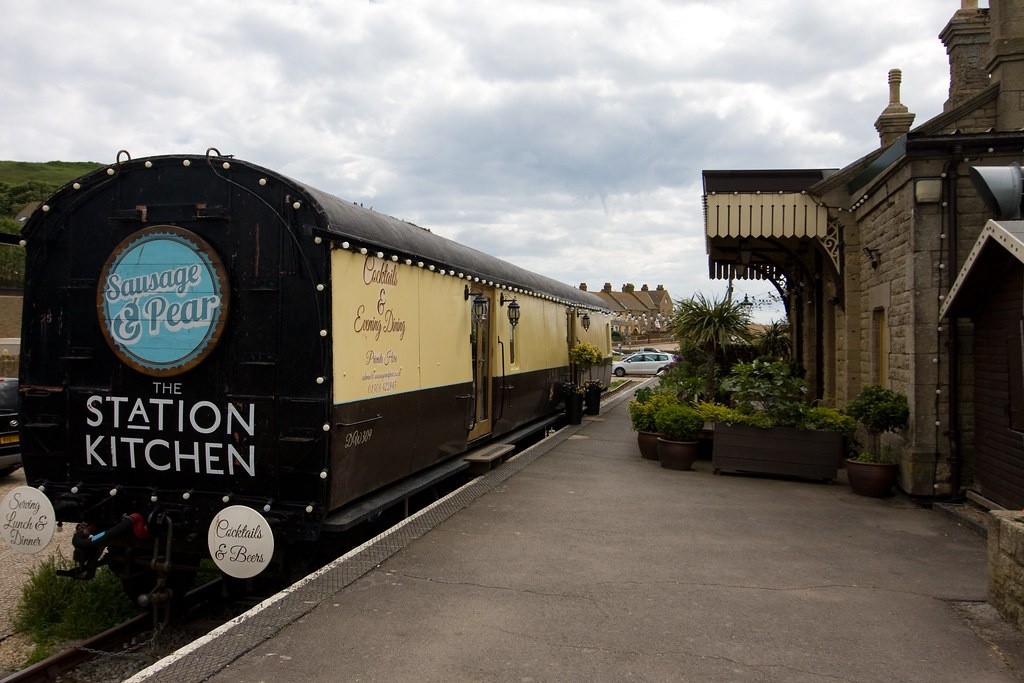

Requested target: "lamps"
[
  {"left": 577, "top": 308, "right": 591, "bottom": 333},
  {"left": 463, "top": 284, "right": 488, "bottom": 324},
  {"left": 499, "top": 292, "right": 520, "bottom": 328}
]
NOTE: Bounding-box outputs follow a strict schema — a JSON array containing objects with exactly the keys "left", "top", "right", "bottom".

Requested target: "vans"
[{"left": 612, "top": 352, "right": 677, "bottom": 377}]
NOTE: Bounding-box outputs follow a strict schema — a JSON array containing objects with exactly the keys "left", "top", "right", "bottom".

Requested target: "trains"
[{"left": 15, "top": 150, "right": 611, "bottom": 615}]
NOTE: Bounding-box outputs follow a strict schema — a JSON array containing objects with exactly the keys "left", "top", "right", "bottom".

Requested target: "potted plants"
[
  {"left": 840, "top": 381, "right": 910, "bottom": 498},
  {"left": 626, "top": 400, "right": 662, "bottom": 461},
  {"left": 692, "top": 390, "right": 859, "bottom": 484},
  {"left": 655, "top": 404, "right": 705, "bottom": 470},
  {"left": 571, "top": 341, "right": 603, "bottom": 371}
]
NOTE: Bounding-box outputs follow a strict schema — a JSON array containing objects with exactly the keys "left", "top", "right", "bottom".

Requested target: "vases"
[
  {"left": 565, "top": 393, "right": 583, "bottom": 425},
  {"left": 586, "top": 389, "right": 601, "bottom": 416}
]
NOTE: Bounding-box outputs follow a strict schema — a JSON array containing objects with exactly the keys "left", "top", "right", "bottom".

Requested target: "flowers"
[
  {"left": 584, "top": 379, "right": 605, "bottom": 393},
  {"left": 565, "top": 383, "right": 585, "bottom": 395}
]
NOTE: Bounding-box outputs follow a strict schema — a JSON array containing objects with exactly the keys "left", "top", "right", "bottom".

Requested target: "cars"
[{"left": 1, "top": 378, "right": 24, "bottom": 479}]
